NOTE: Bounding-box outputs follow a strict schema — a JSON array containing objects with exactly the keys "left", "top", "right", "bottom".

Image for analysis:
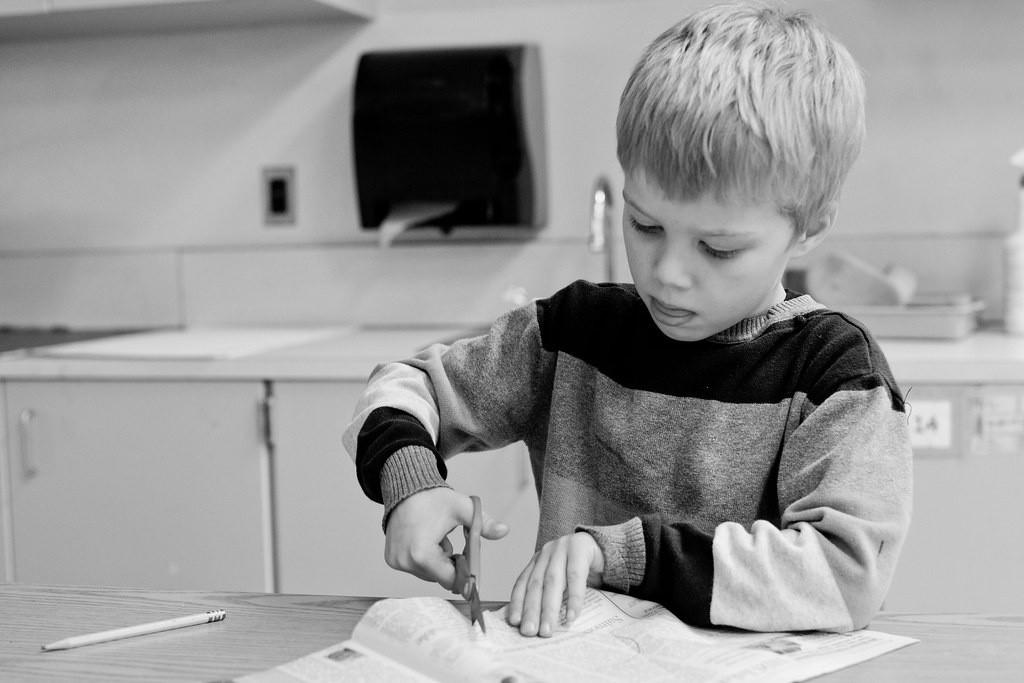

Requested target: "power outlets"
[{"left": 905, "top": 401, "right": 952, "bottom": 449}]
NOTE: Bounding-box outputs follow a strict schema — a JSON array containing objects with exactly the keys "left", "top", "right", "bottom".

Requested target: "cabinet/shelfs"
[{"left": 0, "top": 379, "right": 540, "bottom": 603}]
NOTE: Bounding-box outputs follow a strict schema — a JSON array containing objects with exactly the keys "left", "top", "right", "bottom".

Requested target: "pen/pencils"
[{"left": 39, "top": 608, "right": 226, "bottom": 652}]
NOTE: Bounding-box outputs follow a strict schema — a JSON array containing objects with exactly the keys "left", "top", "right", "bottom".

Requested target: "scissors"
[{"left": 438, "top": 496, "right": 485, "bottom": 635}]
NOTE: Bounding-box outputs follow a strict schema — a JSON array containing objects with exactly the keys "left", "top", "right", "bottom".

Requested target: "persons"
[{"left": 340, "top": 5, "right": 916, "bottom": 634}]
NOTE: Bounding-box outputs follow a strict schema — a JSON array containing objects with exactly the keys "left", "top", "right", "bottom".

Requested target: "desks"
[{"left": 0, "top": 584, "right": 1024, "bottom": 683}]
